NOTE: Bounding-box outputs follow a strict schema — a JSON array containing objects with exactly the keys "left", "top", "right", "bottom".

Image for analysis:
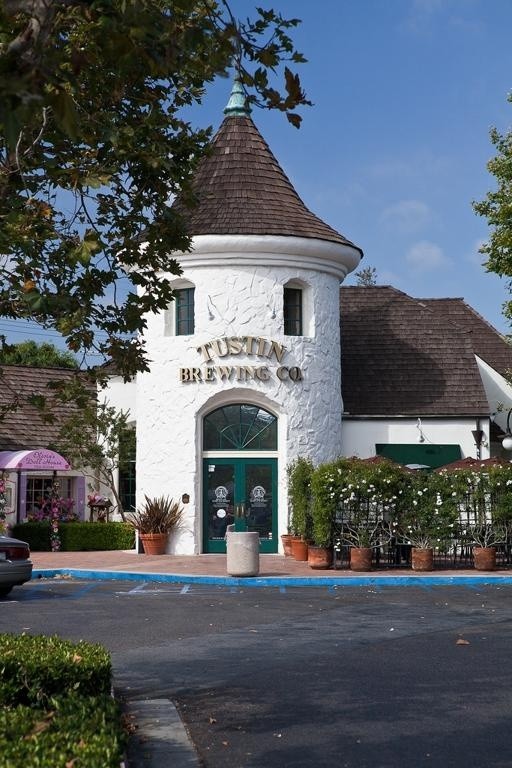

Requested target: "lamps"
[
  {"left": 206, "top": 294, "right": 216, "bottom": 320},
  {"left": 416, "top": 417, "right": 425, "bottom": 441},
  {"left": 266, "top": 292, "right": 277, "bottom": 320}
]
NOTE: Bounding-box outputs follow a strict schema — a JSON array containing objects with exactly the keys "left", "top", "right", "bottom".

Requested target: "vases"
[
  {"left": 471, "top": 547, "right": 496, "bottom": 570},
  {"left": 411, "top": 547, "right": 433, "bottom": 571},
  {"left": 282, "top": 534, "right": 332, "bottom": 570},
  {"left": 348, "top": 547, "right": 374, "bottom": 572}
]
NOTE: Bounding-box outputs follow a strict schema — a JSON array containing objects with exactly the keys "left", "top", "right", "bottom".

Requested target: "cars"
[{"left": 0, "top": 535, "right": 33, "bottom": 597}]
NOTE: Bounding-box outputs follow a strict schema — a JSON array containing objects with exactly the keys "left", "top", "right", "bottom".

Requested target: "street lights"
[{"left": 496, "top": 407, "right": 512, "bottom": 450}]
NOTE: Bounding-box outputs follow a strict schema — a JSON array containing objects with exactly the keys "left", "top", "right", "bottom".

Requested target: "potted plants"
[{"left": 120, "top": 489, "right": 186, "bottom": 555}]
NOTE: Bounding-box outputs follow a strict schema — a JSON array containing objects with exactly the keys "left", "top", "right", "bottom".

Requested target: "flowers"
[{"left": 281, "top": 454, "right": 512, "bottom": 550}]
